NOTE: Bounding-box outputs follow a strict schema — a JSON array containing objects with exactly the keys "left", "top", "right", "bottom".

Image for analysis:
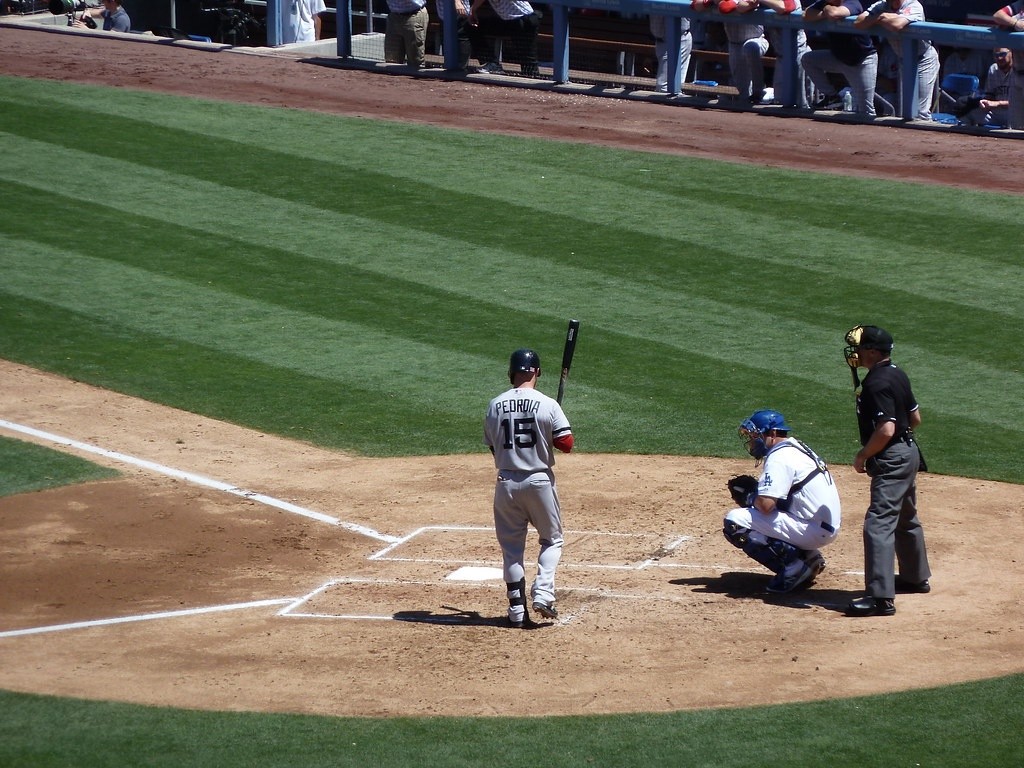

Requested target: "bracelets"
[{"left": 1009, "top": 18, "right": 1017, "bottom": 32}]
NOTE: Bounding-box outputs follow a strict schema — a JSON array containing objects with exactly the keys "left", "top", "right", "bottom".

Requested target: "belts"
[
  {"left": 890, "top": 430, "right": 914, "bottom": 445},
  {"left": 653, "top": 29, "right": 690, "bottom": 42}
]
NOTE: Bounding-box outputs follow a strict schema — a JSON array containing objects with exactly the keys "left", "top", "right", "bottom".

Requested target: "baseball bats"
[{"left": 556, "top": 318, "right": 581, "bottom": 407}]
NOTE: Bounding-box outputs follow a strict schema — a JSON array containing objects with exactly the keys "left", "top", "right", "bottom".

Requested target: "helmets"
[
  {"left": 750, "top": 409, "right": 793, "bottom": 434},
  {"left": 508, "top": 348, "right": 541, "bottom": 377}
]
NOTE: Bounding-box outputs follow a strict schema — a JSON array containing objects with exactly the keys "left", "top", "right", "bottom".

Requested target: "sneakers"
[
  {"left": 813, "top": 95, "right": 843, "bottom": 110},
  {"left": 894, "top": 574, "right": 930, "bottom": 593},
  {"left": 476, "top": 61, "right": 503, "bottom": 74},
  {"left": 848, "top": 596, "right": 896, "bottom": 616},
  {"left": 532, "top": 601, "right": 558, "bottom": 619},
  {"left": 507, "top": 613, "right": 530, "bottom": 628}
]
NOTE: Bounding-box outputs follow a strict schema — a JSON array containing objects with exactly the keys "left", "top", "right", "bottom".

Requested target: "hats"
[{"left": 860, "top": 324, "right": 894, "bottom": 352}]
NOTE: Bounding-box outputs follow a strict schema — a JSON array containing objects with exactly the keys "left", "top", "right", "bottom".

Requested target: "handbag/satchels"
[
  {"left": 944, "top": 90, "right": 996, "bottom": 118},
  {"left": 211, "top": 2, "right": 267, "bottom": 48}
]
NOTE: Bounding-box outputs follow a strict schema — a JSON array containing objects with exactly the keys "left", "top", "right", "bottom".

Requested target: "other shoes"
[{"left": 766, "top": 551, "right": 826, "bottom": 593}]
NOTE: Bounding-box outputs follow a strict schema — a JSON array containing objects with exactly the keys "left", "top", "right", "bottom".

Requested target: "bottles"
[
  {"left": 844, "top": 91, "right": 853, "bottom": 110},
  {"left": 67, "top": 15, "right": 72, "bottom": 27}
]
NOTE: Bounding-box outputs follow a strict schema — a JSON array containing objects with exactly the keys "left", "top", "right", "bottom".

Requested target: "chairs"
[{"left": 931, "top": 73, "right": 980, "bottom": 124}]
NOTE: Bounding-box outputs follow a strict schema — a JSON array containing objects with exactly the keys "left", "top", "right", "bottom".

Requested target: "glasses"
[{"left": 991, "top": 51, "right": 1011, "bottom": 57}]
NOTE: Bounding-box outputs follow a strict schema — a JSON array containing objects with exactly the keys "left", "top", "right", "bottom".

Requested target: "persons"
[
  {"left": 384, "top": 0, "right": 545, "bottom": 76},
  {"left": 844, "top": 324, "right": 933, "bottom": 616},
  {"left": 282, "top": 0, "right": 327, "bottom": 43},
  {"left": 723, "top": 411, "right": 842, "bottom": 591},
  {"left": 483, "top": 350, "right": 574, "bottom": 628},
  {"left": 644, "top": 0, "right": 1024, "bottom": 130},
  {"left": 71, "top": 0, "right": 130, "bottom": 33}
]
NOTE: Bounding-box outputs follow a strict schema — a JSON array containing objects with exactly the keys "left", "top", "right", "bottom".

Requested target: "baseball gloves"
[{"left": 722, "top": 473, "right": 760, "bottom": 508}]
